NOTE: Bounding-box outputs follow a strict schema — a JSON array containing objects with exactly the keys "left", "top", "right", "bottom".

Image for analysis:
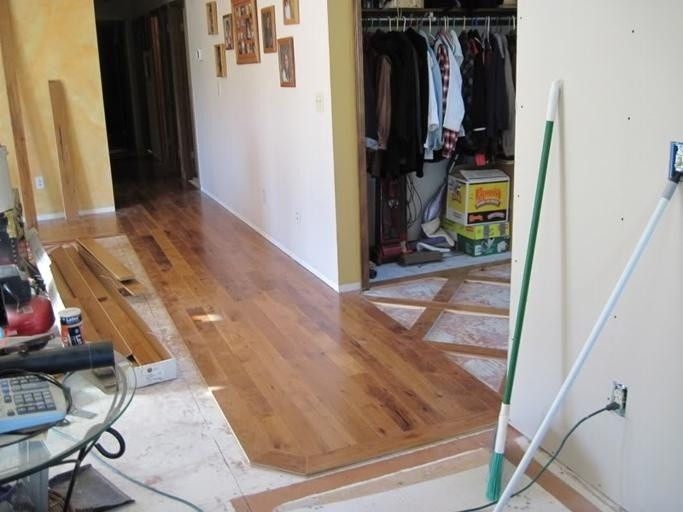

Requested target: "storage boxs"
[
  {"left": 445, "top": 219, "right": 511, "bottom": 256},
  {"left": 443, "top": 170, "right": 510, "bottom": 225}
]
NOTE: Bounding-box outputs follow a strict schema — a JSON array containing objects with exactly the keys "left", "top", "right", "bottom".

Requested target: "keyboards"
[{"left": 1, "top": 376, "right": 67, "bottom": 435}]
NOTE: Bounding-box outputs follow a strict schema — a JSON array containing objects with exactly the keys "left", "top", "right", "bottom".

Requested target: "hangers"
[{"left": 358, "top": 6, "right": 516, "bottom": 46}]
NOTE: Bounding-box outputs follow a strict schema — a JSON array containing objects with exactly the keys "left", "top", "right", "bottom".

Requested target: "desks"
[{"left": 1, "top": 338, "right": 135, "bottom": 512}]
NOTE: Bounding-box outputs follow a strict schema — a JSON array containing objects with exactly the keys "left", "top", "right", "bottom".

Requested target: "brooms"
[{"left": 484, "top": 77, "right": 566, "bottom": 506}]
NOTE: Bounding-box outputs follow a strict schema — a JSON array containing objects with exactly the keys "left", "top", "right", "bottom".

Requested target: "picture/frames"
[
  {"left": 276, "top": 37, "right": 296, "bottom": 86},
  {"left": 260, "top": 5, "right": 277, "bottom": 53},
  {"left": 230, "top": 0, "right": 261, "bottom": 65},
  {"left": 222, "top": 13, "right": 234, "bottom": 50},
  {"left": 282, "top": 0, "right": 299, "bottom": 24},
  {"left": 206, "top": 1, "right": 219, "bottom": 35},
  {"left": 214, "top": 45, "right": 226, "bottom": 77}
]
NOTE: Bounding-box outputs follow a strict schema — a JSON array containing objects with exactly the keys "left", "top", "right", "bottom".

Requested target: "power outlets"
[{"left": 608, "top": 382, "right": 630, "bottom": 415}]
